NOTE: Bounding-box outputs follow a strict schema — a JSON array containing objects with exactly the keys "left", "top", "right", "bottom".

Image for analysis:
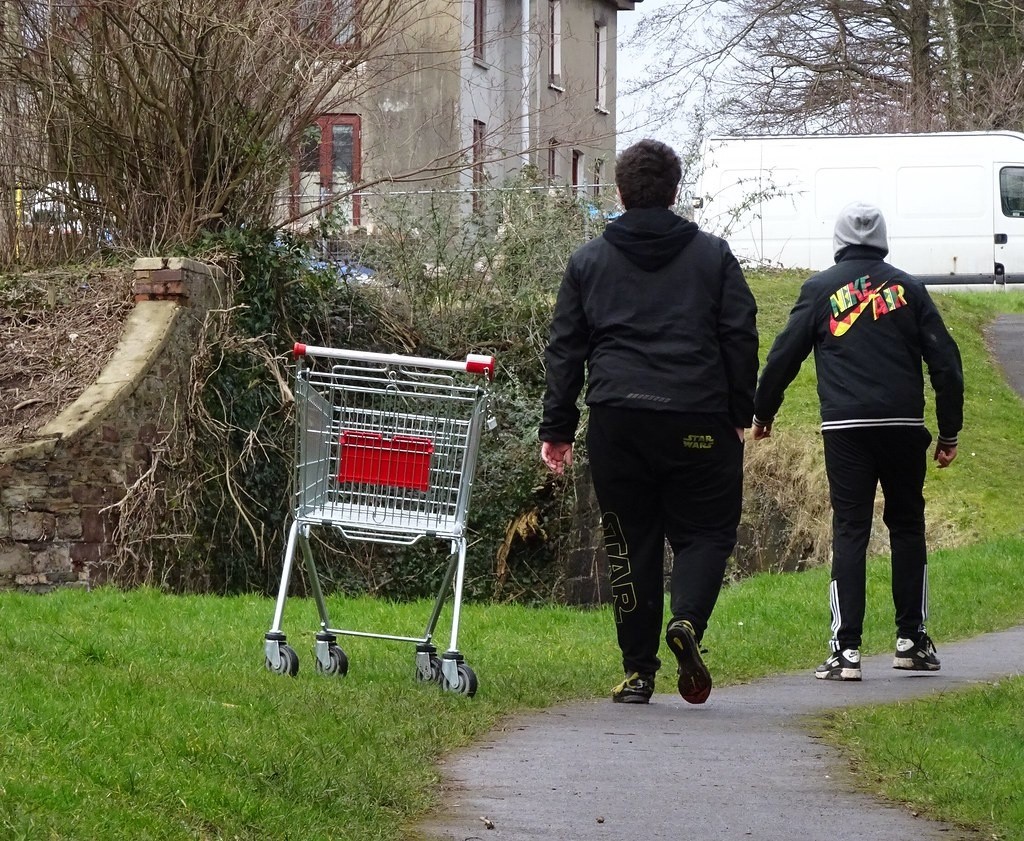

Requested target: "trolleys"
[{"left": 265, "top": 340, "right": 496, "bottom": 698}]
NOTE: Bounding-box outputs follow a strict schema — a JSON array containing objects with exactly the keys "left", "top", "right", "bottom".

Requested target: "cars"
[
  {"left": 23, "top": 206, "right": 91, "bottom": 242},
  {"left": 271, "top": 236, "right": 395, "bottom": 291}
]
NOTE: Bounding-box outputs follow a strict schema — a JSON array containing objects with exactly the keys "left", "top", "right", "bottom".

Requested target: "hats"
[{"left": 833, "top": 202, "right": 888, "bottom": 253}]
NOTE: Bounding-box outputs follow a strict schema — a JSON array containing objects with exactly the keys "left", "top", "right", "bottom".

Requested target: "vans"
[
  {"left": 691, "top": 128, "right": 1023, "bottom": 291},
  {"left": 32, "top": 180, "right": 102, "bottom": 221}
]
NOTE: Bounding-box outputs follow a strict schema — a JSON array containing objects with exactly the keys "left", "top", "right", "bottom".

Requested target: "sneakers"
[
  {"left": 814, "top": 647, "right": 862, "bottom": 681},
  {"left": 891, "top": 624, "right": 941, "bottom": 671},
  {"left": 666, "top": 618, "right": 712, "bottom": 704},
  {"left": 611, "top": 672, "right": 655, "bottom": 703}
]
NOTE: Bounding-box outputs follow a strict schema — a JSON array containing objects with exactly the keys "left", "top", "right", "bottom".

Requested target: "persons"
[
  {"left": 750, "top": 200, "right": 965, "bottom": 680},
  {"left": 538, "top": 140, "right": 759, "bottom": 701}
]
